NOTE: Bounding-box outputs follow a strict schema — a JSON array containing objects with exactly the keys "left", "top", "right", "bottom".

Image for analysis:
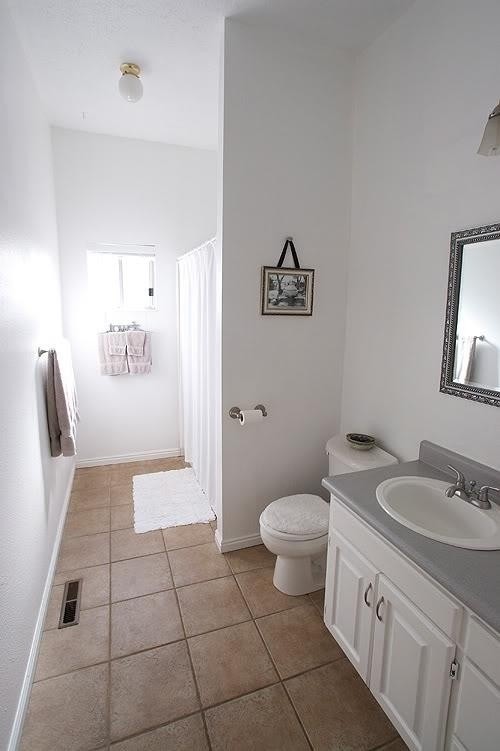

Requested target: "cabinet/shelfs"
[
  {"left": 319, "top": 499, "right": 464, "bottom": 750},
  {"left": 453, "top": 609, "right": 500, "bottom": 750}
]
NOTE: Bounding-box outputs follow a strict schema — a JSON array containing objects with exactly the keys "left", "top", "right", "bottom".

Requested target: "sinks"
[{"left": 375, "top": 475, "right": 500, "bottom": 551}]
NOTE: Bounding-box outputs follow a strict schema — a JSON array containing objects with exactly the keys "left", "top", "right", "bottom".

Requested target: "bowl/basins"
[{"left": 345, "top": 432, "right": 376, "bottom": 451}]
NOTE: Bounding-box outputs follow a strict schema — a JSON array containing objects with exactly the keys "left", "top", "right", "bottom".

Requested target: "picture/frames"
[{"left": 260, "top": 264, "right": 315, "bottom": 319}]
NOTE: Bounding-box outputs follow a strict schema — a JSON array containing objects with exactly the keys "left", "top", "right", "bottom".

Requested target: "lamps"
[{"left": 118, "top": 63, "right": 144, "bottom": 106}]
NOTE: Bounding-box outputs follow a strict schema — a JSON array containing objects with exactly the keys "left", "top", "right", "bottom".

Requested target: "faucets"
[{"left": 445, "top": 481, "right": 480, "bottom": 502}]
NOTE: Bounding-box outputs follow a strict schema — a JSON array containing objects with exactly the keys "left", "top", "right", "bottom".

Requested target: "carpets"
[{"left": 128, "top": 467, "right": 216, "bottom": 538}]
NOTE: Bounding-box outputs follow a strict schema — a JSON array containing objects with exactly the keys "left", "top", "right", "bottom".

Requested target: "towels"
[
  {"left": 44, "top": 348, "right": 83, "bottom": 459},
  {"left": 98, "top": 328, "right": 153, "bottom": 378},
  {"left": 454, "top": 337, "right": 478, "bottom": 385}
]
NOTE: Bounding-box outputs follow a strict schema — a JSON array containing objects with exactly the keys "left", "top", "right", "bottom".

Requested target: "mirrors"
[{"left": 436, "top": 225, "right": 500, "bottom": 409}]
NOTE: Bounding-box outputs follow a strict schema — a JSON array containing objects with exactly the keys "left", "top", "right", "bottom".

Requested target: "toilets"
[{"left": 257, "top": 432, "right": 399, "bottom": 596}]
